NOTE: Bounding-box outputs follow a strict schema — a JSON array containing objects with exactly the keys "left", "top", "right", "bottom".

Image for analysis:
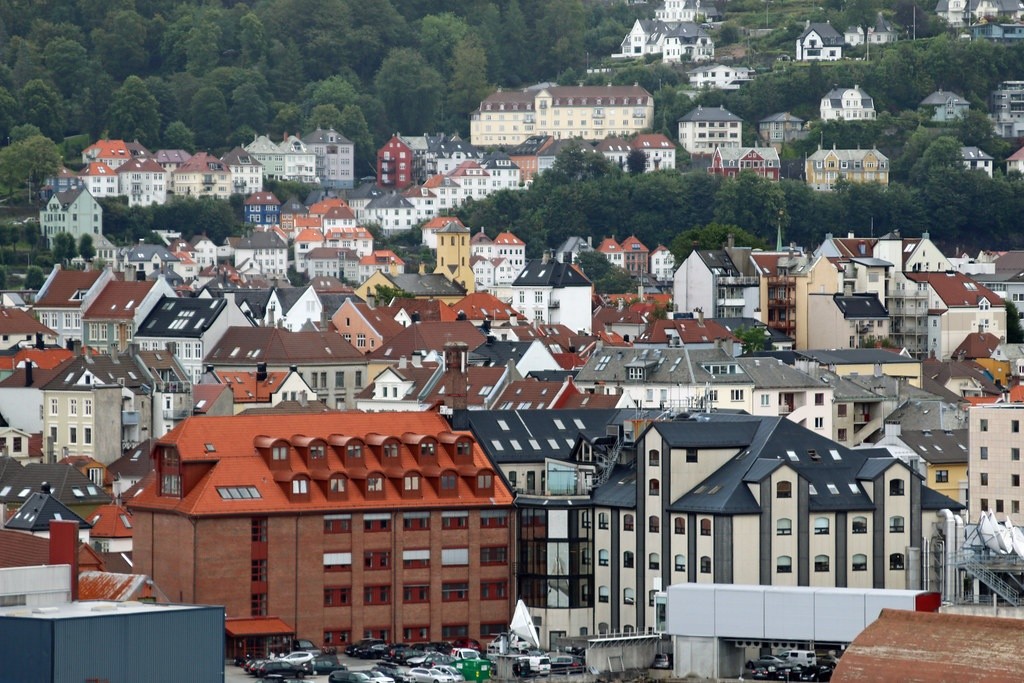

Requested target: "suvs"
[{"left": 372, "top": 661, "right": 417, "bottom": 683}]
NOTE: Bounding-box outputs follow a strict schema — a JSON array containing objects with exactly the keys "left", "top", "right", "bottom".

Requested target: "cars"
[
  {"left": 361, "top": 670, "right": 395, "bottom": 683},
  {"left": 381, "top": 638, "right": 482, "bottom": 666},
  {"left": 354, "top": 639, "right": 386, "bottom": 659},
  {"left": 747, "top": 655, "right": 793, "bottom": 668},
  {"left": 255, "top": 674, "right": 314, "bottom": 683},
  {"left": 653, "top": 654, "right": 672, "bottom": 669},
  {"left": 409, "top": 668, "right": 454, "bottom": 683},
  {"left": 779, "top": 651, "right": 817, "bottom": 667},
  {"left": 495, "top": 627, "right": 585, "bottom": 675},
  {"left": 239, "top": 639, "right": 348, "bottom": 676},
  {"left": 751, "top": 663, "right": 833, "bottom": 682},
  {"left": 328, "top": 670, "right": 373, "bottom": 683},
  {"left": 345, "top": 640, "right": 373, "bottom": 656},
  {"left": 433, "top": 664, "right": 465, "bottom": 683}
]
